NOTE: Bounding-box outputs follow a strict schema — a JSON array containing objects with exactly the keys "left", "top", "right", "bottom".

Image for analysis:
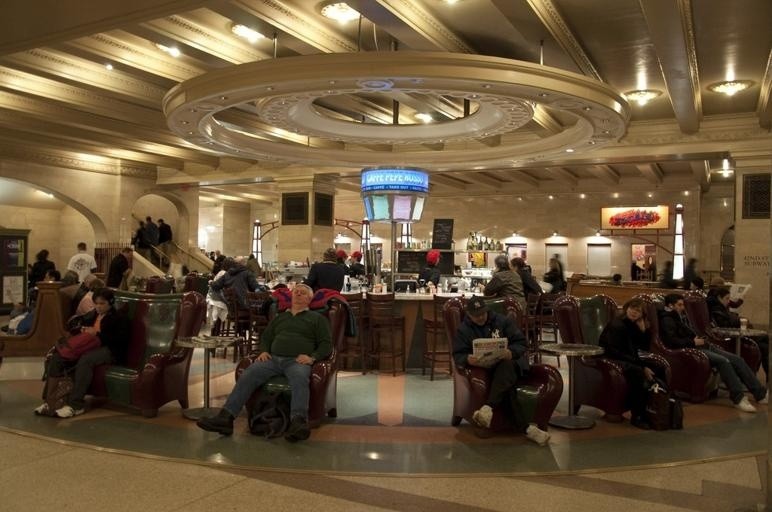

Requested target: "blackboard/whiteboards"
[
  {"left": 432, "top": 218, "right": 454, "bottom": 249},
  {"left": 398, "top": 250, "right": 455, "bottom": 276}
]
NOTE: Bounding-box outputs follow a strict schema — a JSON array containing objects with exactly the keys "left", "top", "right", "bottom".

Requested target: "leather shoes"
[{"left": 630, "top": 414, "right": 652, "bottom": 430}]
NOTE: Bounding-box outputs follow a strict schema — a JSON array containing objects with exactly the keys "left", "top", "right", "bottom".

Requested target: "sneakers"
[
  {"left": 55, "top": 405, "right": 85, "bottom": 418},
  {"left": 758, "top": 389, "right": 768, "bottom": 404},
  {"left": 524, "top": 423, "right": 550, "bottom": 445},
  {"left": 733, "top": 396, "right": 757, "bottom": 413},
  {"left": 33, "top": 403, "right": 48, "bottom": 416},
  {"left": 472, "top": 405, "right": 493, "bottom": 430}
]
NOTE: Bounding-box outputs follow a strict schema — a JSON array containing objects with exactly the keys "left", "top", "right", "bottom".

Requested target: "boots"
[
  {"left": 283, "top": 415, "right": 311, "bottom": 443},
  {"left": 196, "top": 408, "right": 235, "bottom": 437}
]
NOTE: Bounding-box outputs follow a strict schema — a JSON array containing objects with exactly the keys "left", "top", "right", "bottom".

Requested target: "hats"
[
  {"left": 466, "top": 294, "right": 491, "bottom": 316},
  {"left": 336, "top": 249, "right": 348, "bottom": 258},
  {"left": 351, "top": 251, "right": 362, "bottom": 258},
  {"left": 425, "top": 248, "right": 440, "bottom": 265}
]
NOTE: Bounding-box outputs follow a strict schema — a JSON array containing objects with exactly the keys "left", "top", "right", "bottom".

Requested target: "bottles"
[
  {"left": 396, "top": 241, "right": 431, "bottom": 249},
  {"left": 346, "top": 278, "right": 350, "bottom": 292},
  {"left": 466, "top": 231, "right": 501, "bottom": 250}
]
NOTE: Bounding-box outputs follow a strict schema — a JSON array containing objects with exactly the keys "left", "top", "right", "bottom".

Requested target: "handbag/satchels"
[
  {"left": 644, "top": 378, "right": 672, "bottom": 432},
  {"left": 45, "top": 373, "right": 76, "bottom": 418},
  {"left": 247, "top": 393, "right": 290, "bottom": 440},
  {"left": 54, "top": 326, "right": 102, "bottom": 361},
  {"left": 652, "top": 375, "right": 685, "bottom": 431}
]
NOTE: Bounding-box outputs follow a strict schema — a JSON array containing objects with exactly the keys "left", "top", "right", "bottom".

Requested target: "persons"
[
  {"left": 143, "top": 215, "right": 160, "bottom": 248},
  {"left": 450, "top": 293, "right": 551, "bottom": 447},
  {"left": 478, "top": 250, "right": 565, "bottom": 343},
  {"left": 301, "top": 247, "right": 344, "bottom": 294},
  {"left": 131, "top": 220, "right": 152, "bottom": 262},
  {"left": 155, "top": 218, "right": 173, "bottom": 244},
  {"left": 204, "top": 248, "right": 262, "bottom": 338},
  {"left": 419, "top": 248, "right": 443, "bottom": 288},
  {"left": 196, "top": 283, "right": 333, "bottom": 445},
  {"left": 347, "top": 251, "right": 366, "bottom": 276},
  {"left": 0, "top": 240, "right": 133, "bottom": 336},
  {"left": 594, "top": 257, "right": 771, "bottom": 431},
  {"left": 31, "top": 288, "right": 117, "bottom": 420},
  {"left": 336, "top": 249, "right": 355, "bottom": 278}
]
